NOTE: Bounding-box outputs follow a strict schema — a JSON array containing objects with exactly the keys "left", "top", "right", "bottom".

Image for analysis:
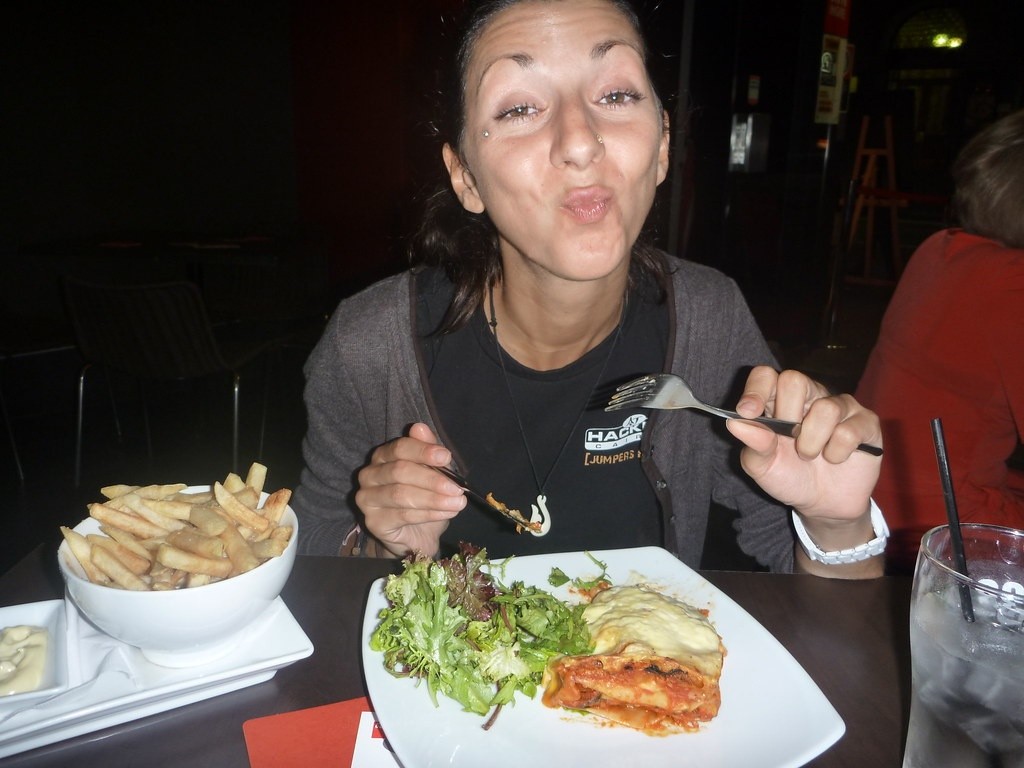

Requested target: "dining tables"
[{"left": 0, "top": 253, "right": 352, "bottom": 491}]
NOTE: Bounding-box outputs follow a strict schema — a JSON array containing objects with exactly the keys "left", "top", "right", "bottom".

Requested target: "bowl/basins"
[{"left": 57, "top": 485, "right": 299, "bottom": 668}]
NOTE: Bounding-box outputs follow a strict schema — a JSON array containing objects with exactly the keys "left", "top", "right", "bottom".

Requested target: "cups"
[{"left": 901, "top": 521, "right": 1024, "bottom": 768}]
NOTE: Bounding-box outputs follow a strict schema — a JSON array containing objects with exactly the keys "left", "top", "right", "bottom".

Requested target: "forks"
[{"left": 604, "top": 373, "right": 884, "bottom": 456}]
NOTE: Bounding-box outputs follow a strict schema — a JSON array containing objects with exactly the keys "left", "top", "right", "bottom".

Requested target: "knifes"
[{"left": 430, "top": 466, "right": 532, "bottom": 531}]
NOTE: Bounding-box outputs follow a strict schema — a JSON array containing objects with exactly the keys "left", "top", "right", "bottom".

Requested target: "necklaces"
[{"left": 487, "top": 271, "right": 631, "bottom": 539}]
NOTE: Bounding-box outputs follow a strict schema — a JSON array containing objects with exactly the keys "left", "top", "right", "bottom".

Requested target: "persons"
[
  {"left": 289, "top": 0, "right": 889, "bottom": 579},
  {"left": 855, "top": 112, "right": 1023, "bottom": 567}
]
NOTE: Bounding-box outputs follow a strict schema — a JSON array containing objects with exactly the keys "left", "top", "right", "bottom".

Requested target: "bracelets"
[
  {"left": 350, "top": 528, "right": 370, "bottom": 558},
  {"left": 791, "top": 497, "right": 889, "bottom": 563}
]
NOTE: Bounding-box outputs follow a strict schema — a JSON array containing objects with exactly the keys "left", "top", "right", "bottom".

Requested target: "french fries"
[{"left": 59, "top": 462, "right": 292, "bottom": 591}]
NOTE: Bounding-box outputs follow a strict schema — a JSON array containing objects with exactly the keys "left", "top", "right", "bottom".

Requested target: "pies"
[{"left": 545, "top": 587, "right": 719, "bottom": 720}]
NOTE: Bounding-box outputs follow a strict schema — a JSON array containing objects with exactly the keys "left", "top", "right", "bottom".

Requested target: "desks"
[
  {"left": 18, "top": 226, "right": 321, "bottom": 447},
  {"left": 0, "top": 542, "right": 1024, "bottom": 768}
]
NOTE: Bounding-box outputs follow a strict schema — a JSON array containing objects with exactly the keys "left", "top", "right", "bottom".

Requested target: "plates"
[
  {"left": 362, "top": 546, "right": 846, "bottom": 768},
  {"left": 0, "top": 600, "right": 80, "bottom": 703},
  {"left": 0, "top": 610, "right": 315, "bottom": 759}
]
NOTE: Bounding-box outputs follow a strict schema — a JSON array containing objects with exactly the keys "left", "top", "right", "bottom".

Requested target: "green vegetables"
[{"left": 367, "top": 542, "right": 595, "bottom": 716}]
